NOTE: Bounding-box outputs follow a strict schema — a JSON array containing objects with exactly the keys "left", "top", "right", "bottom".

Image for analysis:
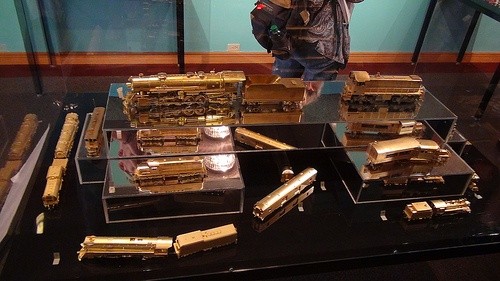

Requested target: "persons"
[{"left": 271, "top": 0, "right": 365, "bottom": 82}]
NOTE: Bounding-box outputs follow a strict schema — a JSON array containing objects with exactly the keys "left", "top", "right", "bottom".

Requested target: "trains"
[
  {"left": 365, "top": 137, "right": 449, "bottom": 177},
  {"left": 233, "top": 127, "right": 293, "bottom": 151},
  {"left": 7, "top": 112, "right": 40, "bottom": 167},
  {"left": 121, "top": 71, "right": 308, "bottom": 113},
  {"left": 132, "top": 156, "right": 207, "bottom": 186},
  {"left": 54, "top": 112, "right": 80, "bottom": 159},
  {"left": 132, "top": 127, "right": 204, "bottom": 147},
  {"left": 42, "top": 158, "right": 70, "bottom": 210},
  {"left": 84, "top": 106, "right": 104, "bottom": 159},
  {"left": 0, "top": 161, "right": 22, "bottom": 214},
  {"left": 253, "top": 184, "right": 314, "bottom": 232},
  {"left": 74, "top": 235, "right": 174, "bottom": 262},
  {"left": 251, "top": 166, "right": 319, "bottom": 221},
  {"left": 403, "top": 198, "right": 472, "bottom": 224},
  {"left": 173, "top": 223, "right": 239, "bottom": 261}
]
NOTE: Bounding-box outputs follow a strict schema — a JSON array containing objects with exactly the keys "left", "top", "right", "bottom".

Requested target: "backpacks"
[{"left": 249, "top": 0, "right": 329, "bottom": 61}]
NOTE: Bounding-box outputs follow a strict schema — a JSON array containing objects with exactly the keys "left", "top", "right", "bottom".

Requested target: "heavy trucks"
[{"left": 340, "top": 69, "right": 423, "bottom": 105}]
